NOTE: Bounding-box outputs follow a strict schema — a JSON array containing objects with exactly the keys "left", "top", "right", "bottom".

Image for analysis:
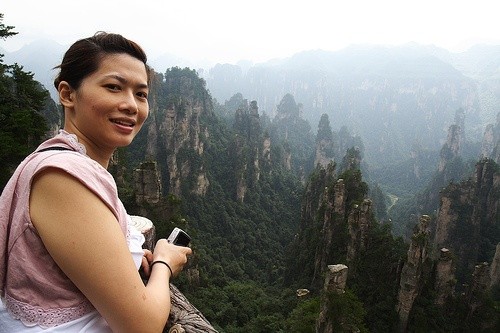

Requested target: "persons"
[{"left": 0, "top": 32, "right": 192, "bottom": 333}]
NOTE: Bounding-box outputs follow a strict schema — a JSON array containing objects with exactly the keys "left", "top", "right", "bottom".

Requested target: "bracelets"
[{"left": 150, "top": 261, "right": 174, "bottom": 278}]
